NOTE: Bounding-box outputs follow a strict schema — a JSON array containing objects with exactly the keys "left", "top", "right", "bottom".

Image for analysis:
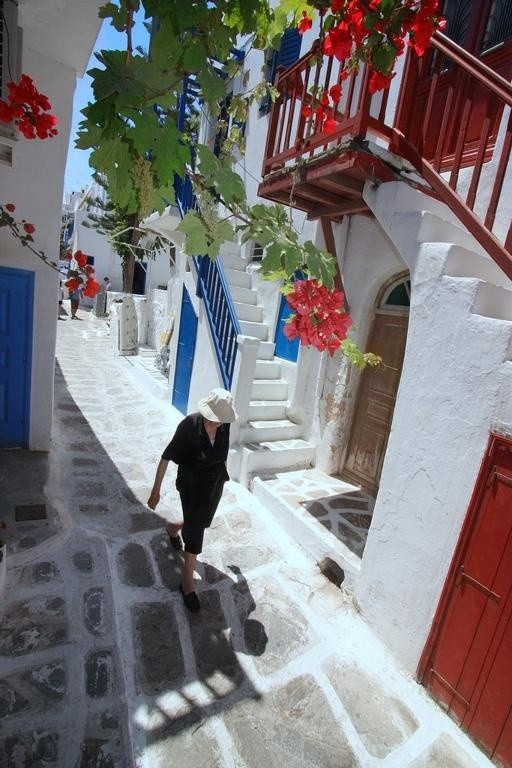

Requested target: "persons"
[
  {"left": 104, "top": 276, "right": 111, "bottom": 313},
  {"left": 57, "top": 279, "right": 63, "bottom": 319},
  {"left": 69, "top": 285, "right": 84, "bottom": 320},
  {"left": 147, "top": 388, "right": 238, "bottom": 612}
]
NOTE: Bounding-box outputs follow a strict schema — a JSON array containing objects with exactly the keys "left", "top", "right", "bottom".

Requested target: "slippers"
[
  {"left": 180, "top": 583, "right": 200, "bottom": 613},
  {"left": 166, "top": 528, "right": 182, "bottom": 549}
]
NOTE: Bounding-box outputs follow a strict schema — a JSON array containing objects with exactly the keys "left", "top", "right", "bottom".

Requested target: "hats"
[{"left": 198, "top": 388, "right": 239, "bottom": 424}]
